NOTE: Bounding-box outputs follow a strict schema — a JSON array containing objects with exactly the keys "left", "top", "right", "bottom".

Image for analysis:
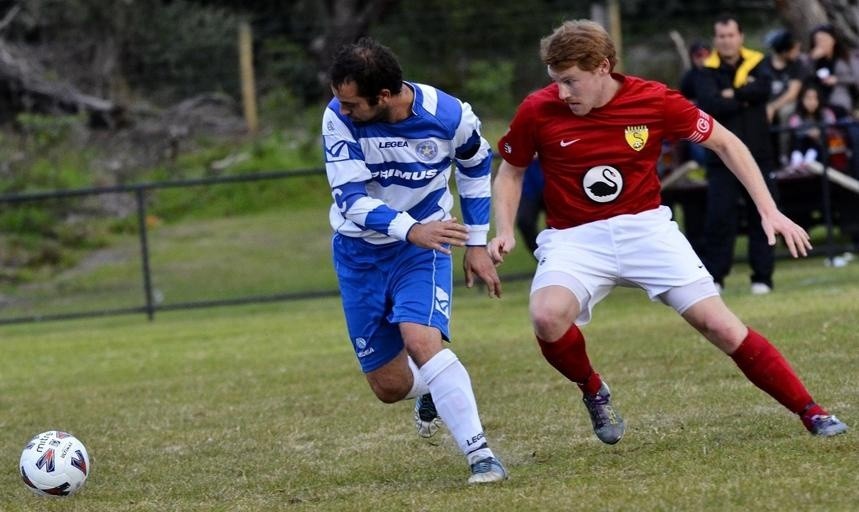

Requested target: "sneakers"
[
  {"left": 583, "top": 381, "right": 624, "bottom": 445},
  {"left": 468, "top": 457, "right": 510, "bottom": 486},
  {"left": 414, "top": 395, "right": 440, "bottom": 437},
  {"left": 811, "top": 414, "right": 848, "bottom": 436},
  {"left": 715, "top": 249, "right": 855, "bottom": 295}
]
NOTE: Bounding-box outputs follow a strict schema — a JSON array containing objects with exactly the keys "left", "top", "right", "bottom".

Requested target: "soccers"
[{"left": 19, "top": 430, "right": 90, "bottom": 498}]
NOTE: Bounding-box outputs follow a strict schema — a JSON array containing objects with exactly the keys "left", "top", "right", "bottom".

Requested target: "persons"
[
  {"left": 511, "top": 88, "right": 549, "bottom": 266},
  {"left": 317, "top": 36, "right": 506, "bottom": 486},
  {"left": 486, "top": 18, "right": 851, "bottom": 445}
]
